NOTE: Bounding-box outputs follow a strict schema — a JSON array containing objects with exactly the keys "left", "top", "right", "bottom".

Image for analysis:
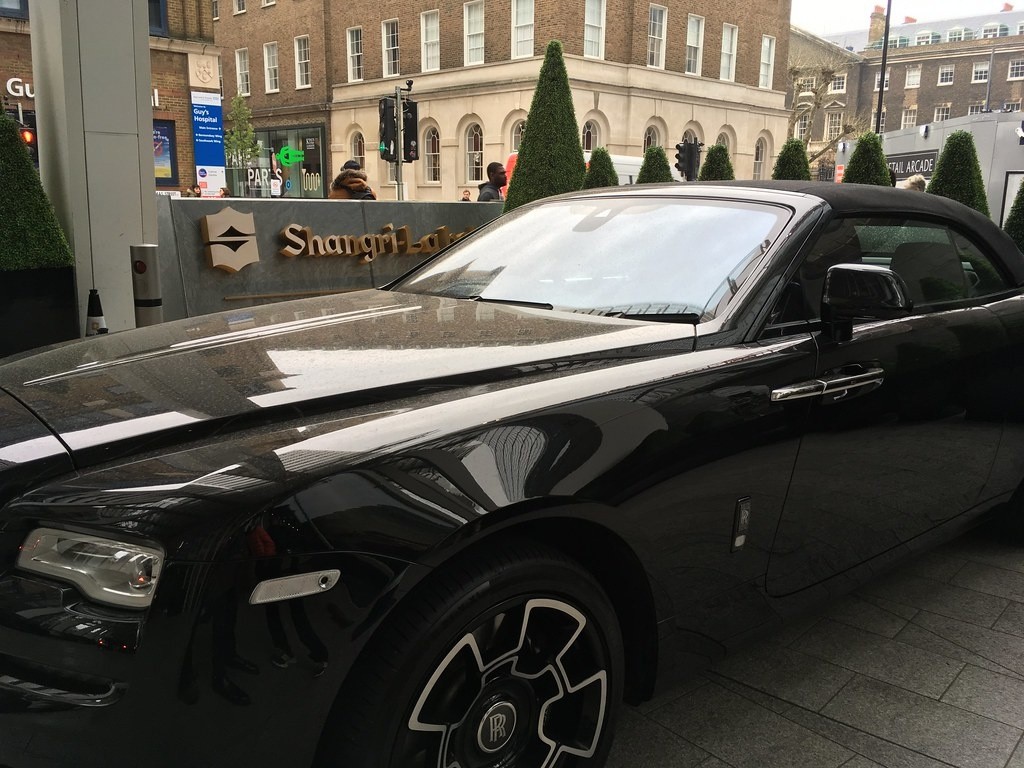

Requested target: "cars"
[{"left": 1, "top": 177, "right": 1024, "bottom": 768}]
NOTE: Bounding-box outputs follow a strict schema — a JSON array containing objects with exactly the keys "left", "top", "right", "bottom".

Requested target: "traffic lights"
[
  {"left": 16, "top": 127, "right": 36, "bottom": 157},
  {"left": 402, "top": 101, "right": 419, "bottom": 162},
  {"left": 377, "top": 98, "right": 396, "bottom": 161}
]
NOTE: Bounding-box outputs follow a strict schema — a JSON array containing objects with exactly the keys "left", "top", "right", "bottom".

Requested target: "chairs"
[{"left": 797, "top": 222, "right": 970, "bottom": 314}]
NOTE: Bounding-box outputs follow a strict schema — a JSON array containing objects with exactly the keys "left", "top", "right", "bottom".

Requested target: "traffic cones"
[{"left": 82, "top": 290, "right": 106, "bottom": 338}]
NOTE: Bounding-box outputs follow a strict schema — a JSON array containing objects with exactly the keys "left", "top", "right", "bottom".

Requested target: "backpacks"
[{"left": 337, "top": 185, "right": 377, "bottom": 200}]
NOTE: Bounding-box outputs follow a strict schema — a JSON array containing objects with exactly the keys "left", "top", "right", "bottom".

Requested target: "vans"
[{"left": 497, "top": 150, "right": 683, "bottom": 212}]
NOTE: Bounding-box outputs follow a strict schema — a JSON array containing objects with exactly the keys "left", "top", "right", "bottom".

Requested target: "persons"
[
  {"left": 458, "top": 190, "right": 471, "bottom": 201},
  {"left": 186, "top": 184, "right": 201, "bottom": 197},
  {"left": 208, "top": 502, "right": 329, "bottom": 707},
  {"left": 476, "top": 161, "right": 507, "bottom": 201},
  {"left": 328, "top": 160, "right": 378, "bottom": 200},
  {"left": 220, "top": 187, "right": 230, "bottom": 197}
]
